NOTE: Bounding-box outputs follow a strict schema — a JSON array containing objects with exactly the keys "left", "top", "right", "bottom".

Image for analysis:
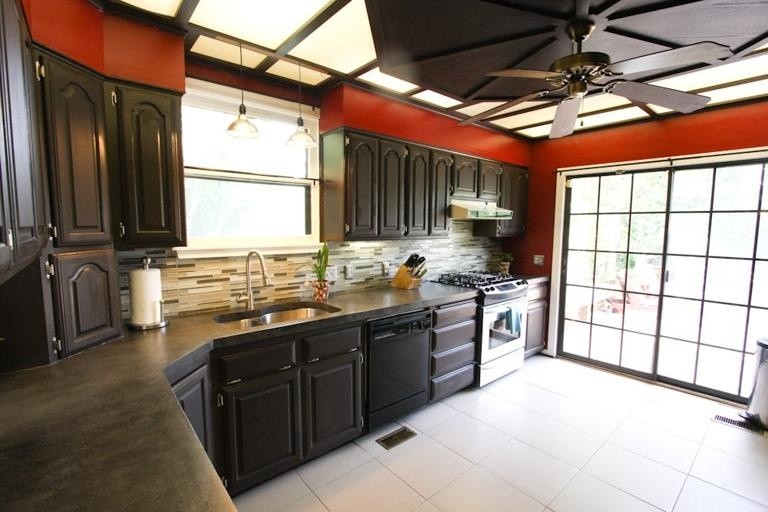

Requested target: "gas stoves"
[{"left": 430, "top": 269, "right": 529, "bottom": 305}]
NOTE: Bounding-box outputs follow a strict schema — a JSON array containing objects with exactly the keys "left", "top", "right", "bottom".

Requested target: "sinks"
[{"left": 212, "top": 301, "right": 342, "bottom": 331}]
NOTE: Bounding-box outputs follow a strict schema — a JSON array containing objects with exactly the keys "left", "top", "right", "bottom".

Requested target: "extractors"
[{"left": 450, "top": 200, "right": 513, "bottom": 220}]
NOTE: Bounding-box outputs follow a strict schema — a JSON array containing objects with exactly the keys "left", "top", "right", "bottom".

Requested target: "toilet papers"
[{"left": 128, "top": 267, "right": 163, "bottom": 326}]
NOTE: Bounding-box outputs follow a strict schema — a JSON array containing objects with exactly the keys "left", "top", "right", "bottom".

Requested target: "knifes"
[{"left": 405, "top": 253, "right": 428, "bottom": 279}]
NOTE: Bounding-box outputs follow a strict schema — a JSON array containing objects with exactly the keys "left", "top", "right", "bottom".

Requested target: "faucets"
[{"left": 246, "top": 250, "right": 271, "bottom": 310}]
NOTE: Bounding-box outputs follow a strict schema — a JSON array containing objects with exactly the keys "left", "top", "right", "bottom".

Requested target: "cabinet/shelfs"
[
  {"left": 405, "top": 136, "right": 454, "bottom": 245},
  {"left": 211, "top": 320, "right": 307, "bottom": 502},
  {"left": 473, "top": 158, "right": 530, "bottom": 242},
  {"left": 520, "top": 274, "right": 552, "bottom": 362},
  {"left": 111, "top": 75, "right": 188, "bottom": 249},
  {"left": 316, "top": 122, "right": 405, "bottom": 243},
  {"left": 451, "top": 151, "right": 503, "bottom": 201},
  {"left": 0, "top": 243, "right": 128, "bottom": 375},
  {"left": 430, "top": 293, "right": 479, "bottom": 410},
  {"left": 164, "top": 337, "right": 232, "bottom": 501},
  {"left": 0, "top": 1, "right": 60, "bottom": 283},
  {"left": 28, "top": 39, "right": 115, "bottom": 252},
  {"left": 292, "top": 310, "right": 366, "bottom": 463}
]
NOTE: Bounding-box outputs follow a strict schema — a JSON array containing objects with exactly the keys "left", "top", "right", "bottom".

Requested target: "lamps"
[
  {"left": 287, "top": 62, "right": 318, "bottom": 151},
  {"left": 225, "top": 42, "right": 259, "bottom": 138}
]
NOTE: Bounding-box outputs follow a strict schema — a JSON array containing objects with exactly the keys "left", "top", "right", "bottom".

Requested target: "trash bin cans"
[{"left": 738, "top": 338, "right": 768, "bottom": 432}]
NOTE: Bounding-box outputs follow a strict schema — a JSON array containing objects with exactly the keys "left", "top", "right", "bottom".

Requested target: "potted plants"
[
  {"left": 497, "top": 250, "right": 513, "bottom": 275},
  {"left": 311, "top": 242, "right": 331, "bottom": 303}
]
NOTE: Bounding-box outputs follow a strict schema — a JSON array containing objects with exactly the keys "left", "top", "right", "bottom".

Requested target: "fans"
[{"left": 454, "top": 12, "right": 738, "bottom": 144}]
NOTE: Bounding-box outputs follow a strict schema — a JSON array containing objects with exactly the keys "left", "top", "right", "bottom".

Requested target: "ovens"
[{"left": 479, "top": 296, "right": 528, "bottom": 388}]
